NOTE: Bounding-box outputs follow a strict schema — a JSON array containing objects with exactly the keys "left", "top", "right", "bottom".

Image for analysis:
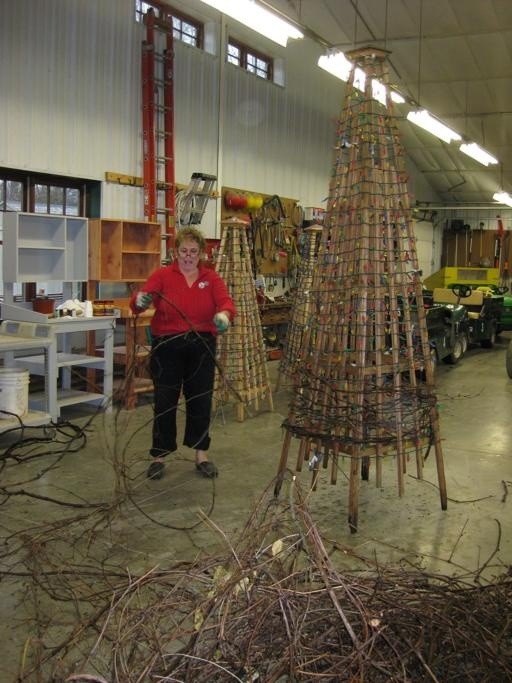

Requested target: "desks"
[{"left": 258, "top": 304, "right": 292, "bottom": 326}]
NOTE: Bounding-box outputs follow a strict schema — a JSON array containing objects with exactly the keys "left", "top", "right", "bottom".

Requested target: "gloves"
[
  {"left": 136, "top": 292, "right": 152, "bottom": 309},
  {"left": 213, "top": 311, "right": 230, "bottom": 332}
]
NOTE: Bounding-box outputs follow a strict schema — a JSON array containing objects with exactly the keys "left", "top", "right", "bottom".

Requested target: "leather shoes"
[
  {"left": 148, "top": 462, "right": 164, "bottom": 479},
  {"left": 196, "top": 462, "right": 218, "bottom": 477}
]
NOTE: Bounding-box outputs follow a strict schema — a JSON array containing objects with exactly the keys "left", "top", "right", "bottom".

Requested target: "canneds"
[
  {"left": 70, "top": 309, "right": 76, "bottom": 317},
  {"left": 55, "top": 309, "right": 63, "bottom": 319}
]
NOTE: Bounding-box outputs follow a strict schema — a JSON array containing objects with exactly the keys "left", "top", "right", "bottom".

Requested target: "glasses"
[{"left": 177, "top": 249, "right": 200, "bottom": 259}]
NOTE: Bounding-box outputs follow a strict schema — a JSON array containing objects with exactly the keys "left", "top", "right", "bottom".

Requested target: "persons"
[{"left": 127, "top": 225, "right": 238, "bottom": 479}]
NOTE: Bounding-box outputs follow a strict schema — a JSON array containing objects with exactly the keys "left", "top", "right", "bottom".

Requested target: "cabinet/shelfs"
[{"left": 0, "top": 212, "right": 162, "bottom": 437}]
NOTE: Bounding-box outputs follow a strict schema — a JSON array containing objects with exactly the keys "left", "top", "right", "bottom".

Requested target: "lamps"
[
  {"left": 406, "top": 3, "right": 463, "bottom": 145},
  {"left": 317, "top": 2, "right": 406, "bottom": 107},
  {"left": 459, "top": 89, "right": 499, "bottom": 166},
  {"left": 202, "top": 1, "right": 306, "bottom": 48}
]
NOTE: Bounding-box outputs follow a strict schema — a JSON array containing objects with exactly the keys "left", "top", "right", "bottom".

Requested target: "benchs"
[{"left": 432, "top": 288, "right": 483, "bottom": 320}]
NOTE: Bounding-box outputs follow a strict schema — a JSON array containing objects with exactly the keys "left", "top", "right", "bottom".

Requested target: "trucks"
[{"left": 384, "top": 266, "right": 512, "bottom": 382}]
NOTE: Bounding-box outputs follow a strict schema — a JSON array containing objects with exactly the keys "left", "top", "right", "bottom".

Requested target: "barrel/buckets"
[{"left": 0, "top": 367, "right": 32, "bottom": 420}]
